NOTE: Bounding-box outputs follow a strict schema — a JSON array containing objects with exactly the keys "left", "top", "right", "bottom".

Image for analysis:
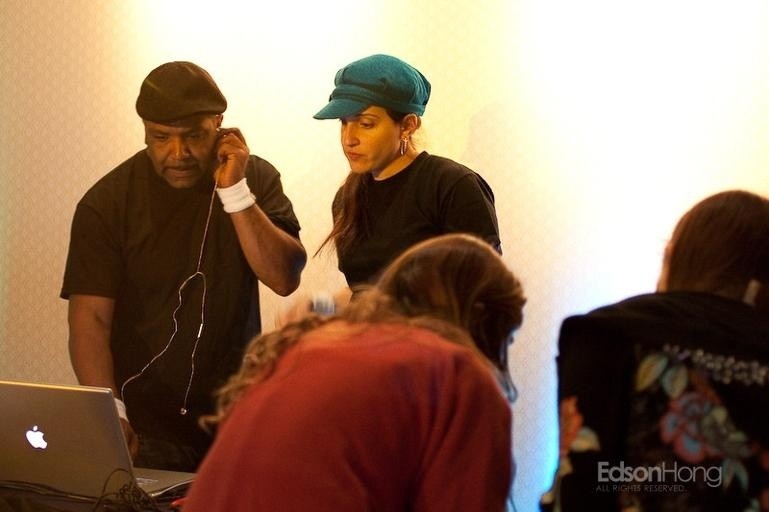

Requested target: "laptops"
[{"left": 0, "top": 381, "right": 198, "bottom": 507}]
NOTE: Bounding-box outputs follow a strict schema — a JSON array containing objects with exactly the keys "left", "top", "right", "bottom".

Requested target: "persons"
[
  {"left": 181, "top": 231, "right": 529, "bottom": 512},
  {"left": 60, "top": 60, "right": 307, "bottom": 473},
  {"left": 535, "top": 188, "right": 768, "bottom": 511},
  {"left": 312, "top": 54, "right": 502, "bottom": 299}
]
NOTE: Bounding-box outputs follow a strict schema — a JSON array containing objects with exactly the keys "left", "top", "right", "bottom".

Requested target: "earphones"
[{"left": 180, "top": 408, "right": 188, "bottom": 415}]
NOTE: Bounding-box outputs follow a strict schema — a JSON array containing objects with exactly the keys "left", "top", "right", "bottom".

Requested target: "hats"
[
  {"left": 136, "top": 61, "right": 227, "bottom": 124},
  {"left": 313, "top": 54, "right": 432, "bottom": 120}
]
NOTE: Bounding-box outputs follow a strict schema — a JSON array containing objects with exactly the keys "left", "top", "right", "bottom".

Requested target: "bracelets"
[
  {"left": 113, "top": 398, "right": 130, "bottom": 423},
  {"left": 216, "top": 178, "right": 257, "bottom": 214}
]
